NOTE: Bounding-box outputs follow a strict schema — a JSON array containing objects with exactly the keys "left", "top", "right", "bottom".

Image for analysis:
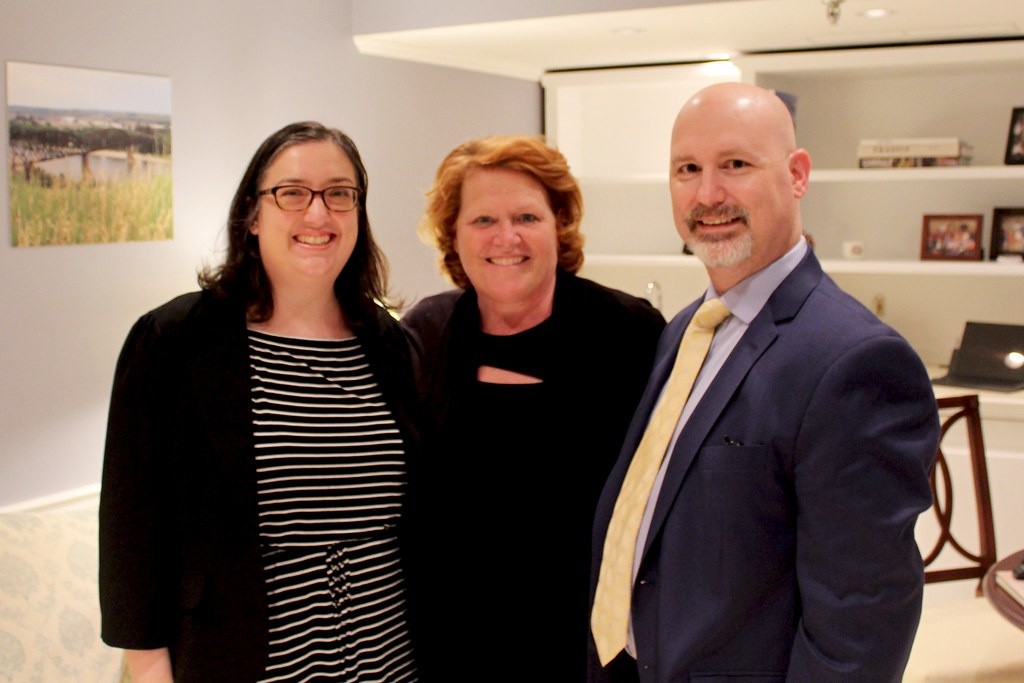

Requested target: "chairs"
[{"left": 904, "top": 396, "right": 1024, "bottom": 683}]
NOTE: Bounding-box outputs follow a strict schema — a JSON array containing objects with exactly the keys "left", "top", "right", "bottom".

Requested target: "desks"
[{"left": 983, "top": 550, "right": 1024, "bottom": 631}]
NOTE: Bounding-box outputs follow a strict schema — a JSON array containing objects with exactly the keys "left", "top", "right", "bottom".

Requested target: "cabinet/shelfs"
[{"left": 735, "top": 40, "right": 1024, "bottom": 556}]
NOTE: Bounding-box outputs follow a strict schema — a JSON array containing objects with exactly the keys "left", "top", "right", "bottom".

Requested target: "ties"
[{"left": 591, "top": 298, "right": 731, "bottom": 667}]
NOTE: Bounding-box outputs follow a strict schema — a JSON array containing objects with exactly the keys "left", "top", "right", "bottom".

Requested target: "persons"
[
  {"left": 97, "top": 121, "right": 434, "bottom": 682},
  {"left": 396, "top": 134, "right": 676, "bottom": 682},
  {"left": 588, "top": 82, "right": 939, "bottom": 682}
]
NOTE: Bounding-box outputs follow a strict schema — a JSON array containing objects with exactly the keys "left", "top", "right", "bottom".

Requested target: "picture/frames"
[
  {"left": 920, "top": 214, "right": 983, "bottom": 262},
  {"left": 1005, "top": 107, "right": 1024, "bottom": 165},
  {"left": 989, "top": 206, "right": 1024, "bottom": 263}
]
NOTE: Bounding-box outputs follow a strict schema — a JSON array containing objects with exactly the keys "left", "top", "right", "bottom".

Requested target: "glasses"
[{"left": 255, "top": 184, "right": 362, "bottom": 212}]
